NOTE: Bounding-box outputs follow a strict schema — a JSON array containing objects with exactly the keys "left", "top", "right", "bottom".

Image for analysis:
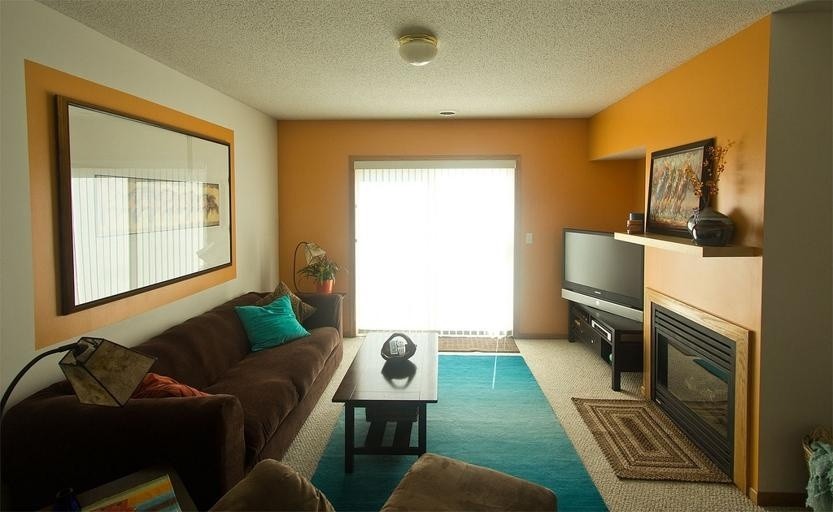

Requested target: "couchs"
[{"left": 0, "top": 292, "right": 342, "bottom": 497}]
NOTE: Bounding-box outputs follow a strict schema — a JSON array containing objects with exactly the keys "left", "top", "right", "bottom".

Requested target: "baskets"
[{"left": 800, "top": 423, "right": 833, "bottom": 482}]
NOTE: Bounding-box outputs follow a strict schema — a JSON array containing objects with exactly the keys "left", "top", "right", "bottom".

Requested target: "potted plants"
[{"left": 296, "top": 258, "right": 346, "bottom": 293}]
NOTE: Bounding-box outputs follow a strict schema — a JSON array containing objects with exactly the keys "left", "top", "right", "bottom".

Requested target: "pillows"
[
  {"left": 256, "top": 280, "right": 317, "bottom": 321},
  {"left": 234, "top": 293, "right": 312, "bottom": 352},
  {"left": 208, "top": 459, "right": 334, "bottom": 512}
]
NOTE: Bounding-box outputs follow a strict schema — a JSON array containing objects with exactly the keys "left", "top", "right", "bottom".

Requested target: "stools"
[{"left": 379, "top": 454, "right": 557, "bottom": 511}]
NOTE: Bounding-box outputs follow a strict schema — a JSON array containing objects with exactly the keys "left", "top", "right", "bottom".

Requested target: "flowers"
[{"left": 684, "top": 139, "right": 736, "bottom": 197}]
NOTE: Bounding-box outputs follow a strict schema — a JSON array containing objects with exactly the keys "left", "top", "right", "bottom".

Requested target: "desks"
[{"left": 332, "top": 332, "right": 438, "bottom": 464}]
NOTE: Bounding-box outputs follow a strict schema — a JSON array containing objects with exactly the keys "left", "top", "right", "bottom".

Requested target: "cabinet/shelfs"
[{"left": 568, "top": 301, "right": 645, "bottom": 391}]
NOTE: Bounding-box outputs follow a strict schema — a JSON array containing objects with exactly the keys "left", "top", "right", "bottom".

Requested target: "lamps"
[
  {"left": 293, "top": 241, "right": 327, "bottom": 293},
  {"left": 398, "top": 33, "right": 437, "bottom": 67},
  {"left": 0, "top": 336, "right": 155, "bottom": 419}
]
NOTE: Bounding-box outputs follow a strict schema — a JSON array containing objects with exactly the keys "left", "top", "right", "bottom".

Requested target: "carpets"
[
  {"left": 571, "top": 395, "right": 732, "bottom": 484},
  {"left": 439, "top": 336, "right": 520, "bottom": 352},
  {"left": 312, "top": 354, "right": 608, "bottom": 512}
]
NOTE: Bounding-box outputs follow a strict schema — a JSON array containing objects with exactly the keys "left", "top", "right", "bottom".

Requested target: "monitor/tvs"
[{"left": 562, "top": 228, "right": 644, "bottom": 311}]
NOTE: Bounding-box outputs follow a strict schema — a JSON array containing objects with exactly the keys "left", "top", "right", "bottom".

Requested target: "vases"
[{"left": 688, "top": 207, "right": 737, "bottom": 244}]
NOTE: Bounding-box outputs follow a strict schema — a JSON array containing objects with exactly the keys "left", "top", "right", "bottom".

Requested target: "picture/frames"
[
  {"left": 52, "top": 93, "right": 232, "bottom": 316},
  {"left": 647, "top": 137, "right": 714, "bottom": 237}
]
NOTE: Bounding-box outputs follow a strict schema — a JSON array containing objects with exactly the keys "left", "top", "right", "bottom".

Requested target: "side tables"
[{"left": 36, "top": 466, "right": 200, "bottom": 512}]
